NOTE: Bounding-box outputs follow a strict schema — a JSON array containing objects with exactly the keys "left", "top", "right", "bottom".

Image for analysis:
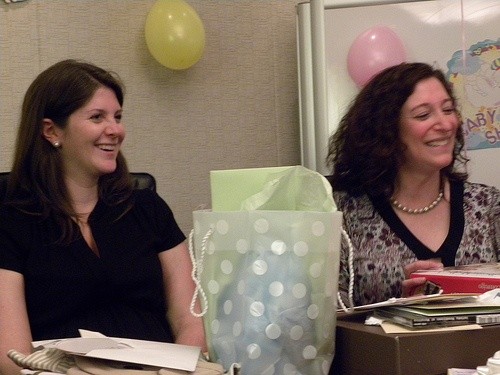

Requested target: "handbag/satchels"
[{"left": 188, "top": 208, "right": 355, "bottom": 375}]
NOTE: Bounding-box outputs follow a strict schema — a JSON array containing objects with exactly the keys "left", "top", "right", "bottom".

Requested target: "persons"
[
  {"left": 324, "top": 62, "right": 500, "bottom": 324},
  {"left": 0, "top": 58, "right": 207, "bottom": 375}
]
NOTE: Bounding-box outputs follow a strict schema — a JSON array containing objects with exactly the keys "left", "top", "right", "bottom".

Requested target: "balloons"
[
  {"left": 143, "top": 0, "right": 208, "bottom": 71},
  {"left": 347, "top": 26, "right": 406, "bottom": 88}
]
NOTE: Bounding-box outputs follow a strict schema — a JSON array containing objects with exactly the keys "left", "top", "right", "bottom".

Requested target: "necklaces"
[{"left": 389, "top": 192, "right": 445, "bottom": 213}]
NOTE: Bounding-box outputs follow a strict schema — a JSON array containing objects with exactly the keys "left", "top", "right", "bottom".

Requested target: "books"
[{"left": 382, "top": 295, "right": 500, "bottom": 329}]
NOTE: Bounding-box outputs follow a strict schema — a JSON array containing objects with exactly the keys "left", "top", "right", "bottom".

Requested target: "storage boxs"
[
  {"left": 327, "top": 312, "right": 500, "bottom": 375},
  {"left": 410, "top": 263, "right": 500, "bottom": 297}
]
